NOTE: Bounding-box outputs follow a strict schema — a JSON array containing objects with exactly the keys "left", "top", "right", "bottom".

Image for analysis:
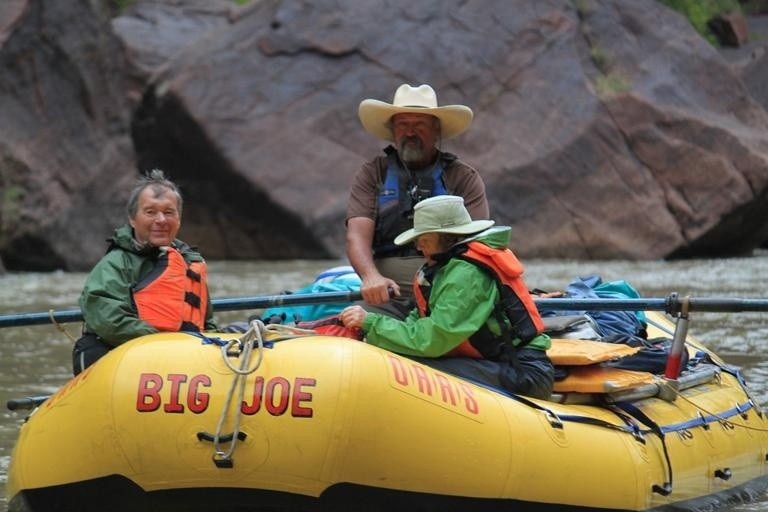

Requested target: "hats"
[
  {"left": 358, "top": 84, "right": 473, "bottom": 143},
  {"left": 393, "top": 196, "right": 495, "bottom": 246}
]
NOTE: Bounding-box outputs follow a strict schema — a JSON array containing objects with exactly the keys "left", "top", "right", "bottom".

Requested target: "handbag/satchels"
[{"left": 597, "top": 333, "right": 689, "bottom": 373}]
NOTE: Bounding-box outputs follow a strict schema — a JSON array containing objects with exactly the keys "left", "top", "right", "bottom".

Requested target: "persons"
[
  {"left": 70, "top": 169, "right": 223, "bottom": 379},
  {"left": 335, "top": 193, "right": 557, "bottom": 402},
  {"left": 345, "top": 83, "right": 494, "bottom": 319}
]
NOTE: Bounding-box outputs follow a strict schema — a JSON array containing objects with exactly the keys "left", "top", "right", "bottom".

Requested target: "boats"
[{"left": 5, "top": 297, "right": 768, "bottom": 511}]
